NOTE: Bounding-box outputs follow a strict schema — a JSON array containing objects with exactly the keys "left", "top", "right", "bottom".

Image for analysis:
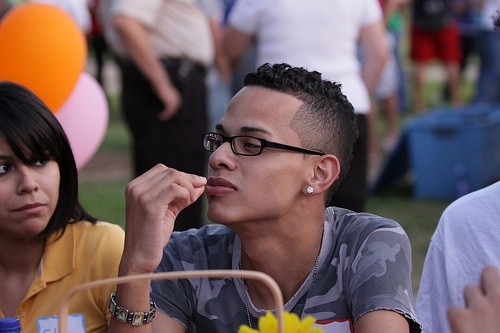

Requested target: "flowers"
[{"left": 237, "top": 309, "right": 324, "bottom": 333}]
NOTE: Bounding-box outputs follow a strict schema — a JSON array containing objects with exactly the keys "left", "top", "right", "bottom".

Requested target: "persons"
[
  {"left": 410, "top": 0, "right": 461, "bottom": 110},
  {"left": 195, "top": 0, "right": 232, "bottom": 161},
  {"left": 415, "top": 180, "right": 500, "bottom": 333},
  {"left": 100, "top": 0, "right": 217, "bottom": 231},
  {"left": 223, "top": 0, "right": 388, "bottom": 208},
  {"left": 21, "top": 0, "right": 105, "bottom": 90},
  {"left": 109, "top": 63, "right": 423, "bottom": 333},
  {"left": 0, "top": 81, "right": 125, "bottom": 333},
  {"left": 357, "top": 0, "right": 408, "bottom": 138},
  {"left": 440, "top": 0, "right": 500, "bottom": 104}
]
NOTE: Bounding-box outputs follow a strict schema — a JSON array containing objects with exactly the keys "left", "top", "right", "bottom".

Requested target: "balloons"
[
  {"left": 0, "top": 3, "right": 87, "bottom": 112},
  {"left": 54, "top": 72, "right": 108, "bottom": 169}
]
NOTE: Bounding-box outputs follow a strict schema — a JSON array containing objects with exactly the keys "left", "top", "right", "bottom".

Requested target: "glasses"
[{"left": 201, "top": 132, "right": 324, "bottom": 156}]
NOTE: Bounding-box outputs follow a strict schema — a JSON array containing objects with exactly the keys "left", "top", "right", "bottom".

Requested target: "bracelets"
[{"left": 108, "top": 290, "right": 157, "bottom": 326}]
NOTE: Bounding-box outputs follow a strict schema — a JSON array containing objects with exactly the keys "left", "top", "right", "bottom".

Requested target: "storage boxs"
[{"left": 404, "top": 103, "right": 500, "bottom": 200}]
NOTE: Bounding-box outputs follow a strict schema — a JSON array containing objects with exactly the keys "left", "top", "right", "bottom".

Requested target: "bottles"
[{"left": 0, "top": 316, "right": 21, "bottom": 333}]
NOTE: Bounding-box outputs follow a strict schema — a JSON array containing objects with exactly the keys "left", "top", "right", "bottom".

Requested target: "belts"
[{"left": 123, "top": 57, "right": 206, "bottom": 77}]
